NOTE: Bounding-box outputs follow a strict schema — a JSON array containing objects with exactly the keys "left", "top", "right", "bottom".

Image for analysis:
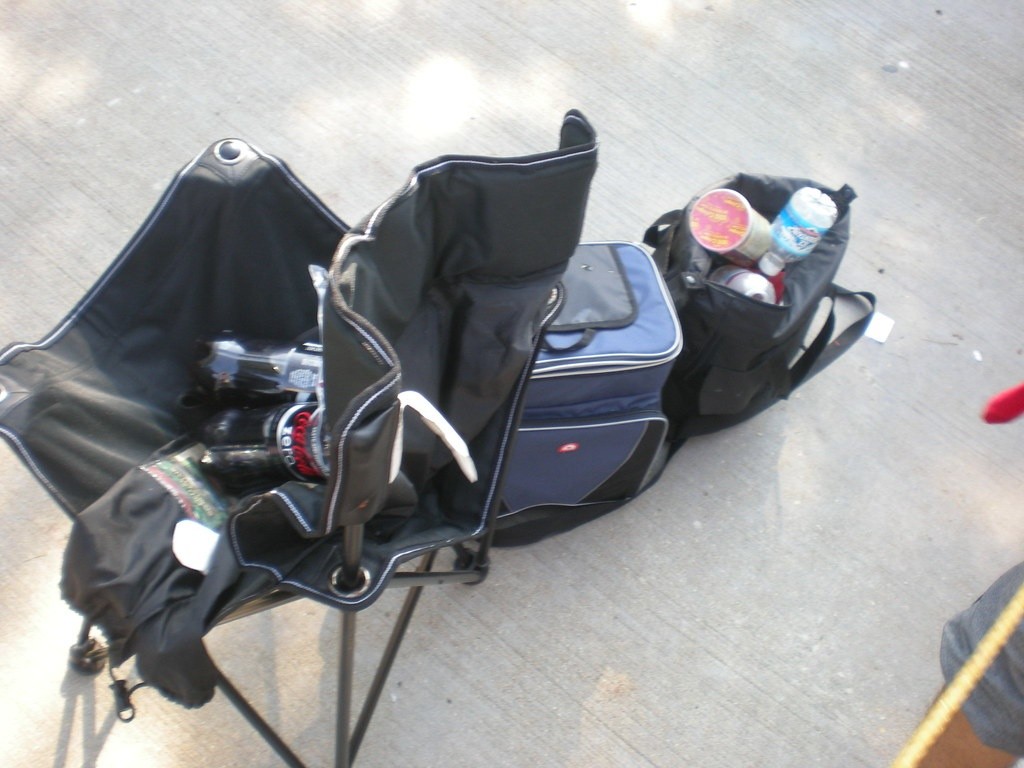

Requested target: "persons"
[{"left": 915, "top": 557, "right": 1024, "bottom": 767}]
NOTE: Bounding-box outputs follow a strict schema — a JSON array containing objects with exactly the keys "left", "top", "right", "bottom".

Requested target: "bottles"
[
  {"left": 760, "top": 187, "right": 838, "bottom": 276},
  {"left": 709, "top": 265, "right": 776, "bottom": 304},
  {"left": 200, "top": 405, "right": 330, "bottom": 498},
  {"left": 197, "top": 328, "right": 324, "bottom": 402}
]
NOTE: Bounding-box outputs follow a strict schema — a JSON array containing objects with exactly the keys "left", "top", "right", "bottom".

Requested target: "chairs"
[{"left": 0, "top": 111, "right": 600, "bottom": 768}]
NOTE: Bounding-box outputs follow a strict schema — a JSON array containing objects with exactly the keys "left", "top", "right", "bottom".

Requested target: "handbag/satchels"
[
  {"left": 491, "top": 243, "right": 685, "bottom": 532},
  {"left": 641, "top": 169, "right": 877, "bottom": 446}
]
